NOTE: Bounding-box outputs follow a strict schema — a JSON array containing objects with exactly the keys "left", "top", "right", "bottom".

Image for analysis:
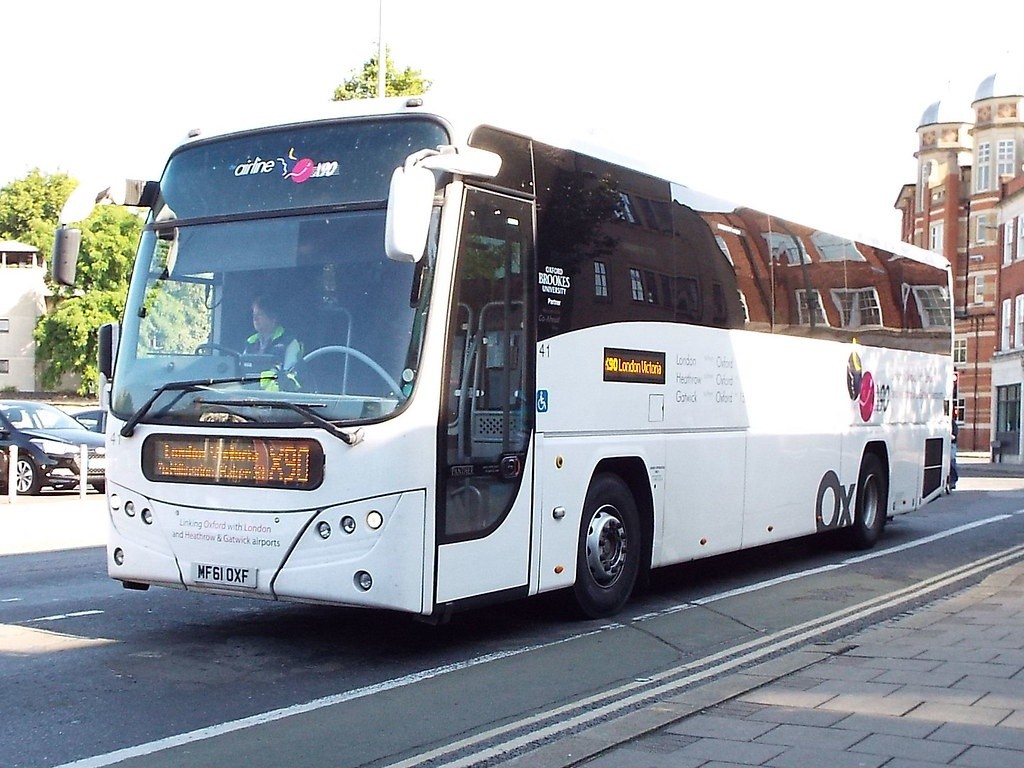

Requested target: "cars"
[
  {"left": 1, "top": 400, "right": 105, "bottom": 493},
  {"left": 60, "top": 408, "right": 108, "bottom": 434}
]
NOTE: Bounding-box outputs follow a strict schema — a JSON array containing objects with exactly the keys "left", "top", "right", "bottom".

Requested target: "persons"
[
  {"left": 241, "top": 294, "right": 303, "bottom": 392},
  {"left": 950, "top": 419, "right": 959, "bottom": 490}
]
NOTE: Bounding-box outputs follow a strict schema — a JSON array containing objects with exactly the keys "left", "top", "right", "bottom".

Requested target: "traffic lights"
[
  {"left": 953, "top": 372, "right": 959, "bottom": 398},
  {"left": 952, "top": 400, "right": 964, "bottom": 422}
]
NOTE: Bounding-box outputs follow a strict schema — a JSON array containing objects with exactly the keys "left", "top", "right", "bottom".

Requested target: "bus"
[{"left": 54, "top": 96, "right": 955, "bottom": 616}]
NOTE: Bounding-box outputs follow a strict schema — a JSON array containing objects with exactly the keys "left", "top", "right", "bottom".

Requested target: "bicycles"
[{"left": 945, "top": 433, "right": 958, "bottom": 495}]
